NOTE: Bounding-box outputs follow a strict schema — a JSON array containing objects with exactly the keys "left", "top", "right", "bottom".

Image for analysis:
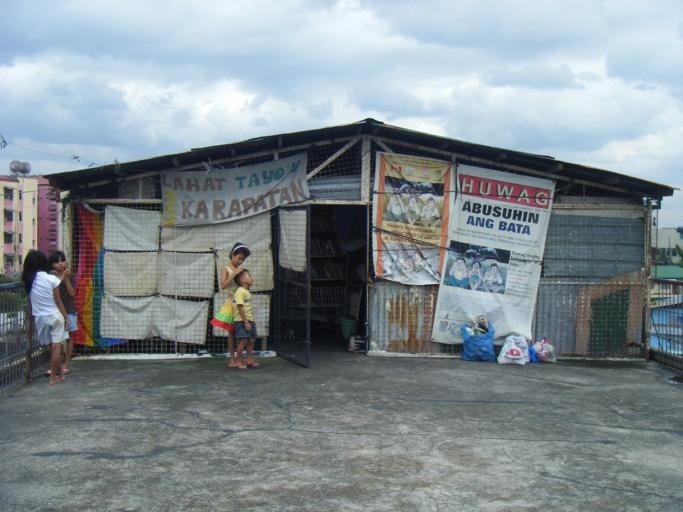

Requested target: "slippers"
[
  {"left": 236, "top": 363, "right": 249, "bottom": 371},
  {"left": 247, "top": 360, "right": 260, "bottom": 368},
  {"left": 44, "top": 367, "right": 70, "bottom": 385},
  {"left": 228, "top": 361, "right": 243, "bottom": 368}
]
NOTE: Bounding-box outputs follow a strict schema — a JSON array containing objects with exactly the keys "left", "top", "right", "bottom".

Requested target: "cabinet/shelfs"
[{"left": 280, "top": 208, "right": 351, "bottom": 343}]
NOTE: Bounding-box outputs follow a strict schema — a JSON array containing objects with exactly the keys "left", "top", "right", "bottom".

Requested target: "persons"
[
  {"left": 209, "top": 242, "right": 243, "bottom": 368},
  {"left": 383, "top": 192, "right": 441, "bottom": 229},
  {"left": 42, "top": 251, "right": 78, "bottom": 376},
  {"left": 21, "top": 249, "right": 70, "bottom": 385},
  {"left": 447, "top": 258, "right": 502, "bottom": 294},
  {"left": 232, "top": 269, "right": 260, "bottom": 370}
]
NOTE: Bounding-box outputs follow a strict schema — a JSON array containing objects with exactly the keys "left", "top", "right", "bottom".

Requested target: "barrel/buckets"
[{"left": 340, "top": 320, "right": 359, "bottom": 341}]
